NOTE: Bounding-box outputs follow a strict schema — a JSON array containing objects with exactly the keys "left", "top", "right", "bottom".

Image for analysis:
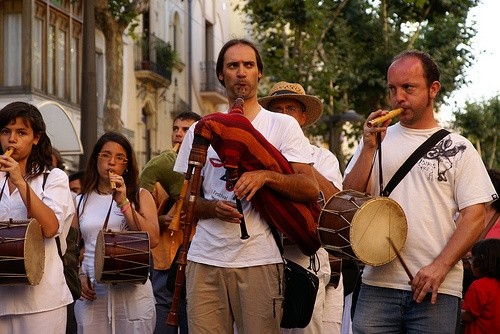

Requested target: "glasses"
[
  {"left": 98, "top": 153, "right": 128, "bottom": 164},
  {"left": 466, "top": 255, "right": 482, "bottom": 264}
]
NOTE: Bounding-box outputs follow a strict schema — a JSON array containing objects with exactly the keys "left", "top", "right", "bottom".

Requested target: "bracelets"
[
  {"left": 120, "top": 202, "right": 131, "bottom": 214},
  {"left": 77, "top": 272, "right": 88, "bottom": 279},
  {"left": 116, "top": 198, "right": 129, "bottom": 208}
]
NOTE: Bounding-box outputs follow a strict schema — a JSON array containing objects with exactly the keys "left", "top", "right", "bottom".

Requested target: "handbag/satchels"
[
  {"left": 61, "top": 225, "right": 82, "bottom": 300},
  {"left": 280, "top": 258, "right": 320, "bottom": 329},
  {"left": 341, "top": 259, "right": 360, "bottom": 297}
]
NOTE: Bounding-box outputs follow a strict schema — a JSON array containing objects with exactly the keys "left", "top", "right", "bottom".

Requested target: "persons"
[
  {"left": 48, "top": 147, "right": 86, "bottom": 334},
  {"left": 342, "top": 49, "right": 499, "bottom": 334},
  {"left": 258, "top": 81, "right": 343, "bottom": 334},
  {"left": 455, "top": 169, "right": 500, "bottom": 301},
  {"left": 137, "top": 111, "right": 204, "bottom": 334},
  {"left": 0, "top": 101, "right": 75, "bottom": 334},
  {"left": 63, "top": 132, "right": 159, "bottom": 334},
  {"left": 460, "top": 238, "right": 500, "bottom": 334},
  {"left": 173, "top": 39, "right": 320, "bottom": 334}
]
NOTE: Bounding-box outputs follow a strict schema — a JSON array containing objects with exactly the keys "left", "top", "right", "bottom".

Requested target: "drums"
[
  {"left": 317, "top": 189, "right": 408, "bottom": 266},
  {"left": 94, "top": 229, "right": 151, "bottom": 285},
  {"left": 462, "top": 257, "right": 477, "bottom": 298},
  {"left": 326, "top": 253, "right": 343, "bottom": 290},
  {"left": 0, "top": 218, "right": 46, "bottom": 286}
]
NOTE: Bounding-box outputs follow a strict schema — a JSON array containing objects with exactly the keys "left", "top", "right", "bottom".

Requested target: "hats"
[{"left": 258, "top": 81, "right": 324, "bottom": 127}]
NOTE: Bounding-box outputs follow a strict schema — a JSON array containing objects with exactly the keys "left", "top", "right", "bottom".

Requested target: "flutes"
[
  {"left": 367, "top": 107, "right": 404, "bottom": 127},
  {"left": 225, "top": 85, "right": 244, "bottom": 181},
  {"left": 234, "top": 187, "right": 250, "bottom": 240},
  {"left": 173, "top": 142, "right": 182, "bottom": 151},
  {"left": 165, "top": 165, "right": 202, "bottom": 327}
]
